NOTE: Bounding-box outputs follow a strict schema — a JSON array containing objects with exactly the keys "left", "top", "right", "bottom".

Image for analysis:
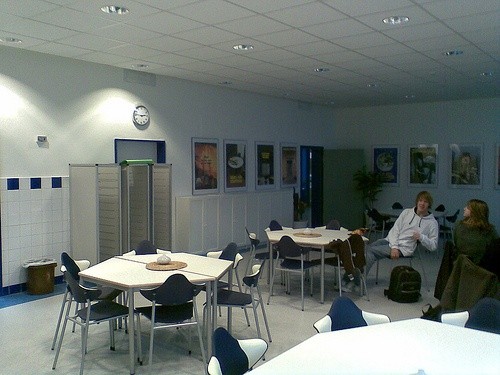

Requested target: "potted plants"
[{"left": 351, "top": 166, "right": 385, "bottom": 228}]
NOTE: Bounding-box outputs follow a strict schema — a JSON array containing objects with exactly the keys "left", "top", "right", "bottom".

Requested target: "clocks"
[{"left": 134, "top": 105, "right": 149, "bottom": 126}]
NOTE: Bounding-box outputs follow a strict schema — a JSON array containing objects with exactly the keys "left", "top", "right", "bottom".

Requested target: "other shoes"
[
  {"left": 333, "top": 280, "right": 347, "bottom": 290},
  {"left": 340, "top": 283, "right": 357, "bottom": 293}
]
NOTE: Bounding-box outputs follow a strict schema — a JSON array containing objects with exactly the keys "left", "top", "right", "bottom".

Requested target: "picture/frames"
[
  {"left": 407, "top": 143, "right": 438, "bottom": 188},
  {"left": 373, "top": 144, "right": 400, "bottom": 187},
  {"left": 191, "top": 137, "right": 219, "bottom": 195},
  {"left": 280, "top": 142, "right": 300, "bottom": 188},
  {"left": 449, "top": 143, "right": 484, "bottom": 189},
  {"left": 223, "top": 139, "right": 248, "bottom": 193},
  {"left": 255, "top": 141, "right": 280, "bottom": 190}
]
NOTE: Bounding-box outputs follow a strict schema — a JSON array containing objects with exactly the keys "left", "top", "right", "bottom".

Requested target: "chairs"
[{"left": 51, "top": 202, "right": 500, "bottom": 375}]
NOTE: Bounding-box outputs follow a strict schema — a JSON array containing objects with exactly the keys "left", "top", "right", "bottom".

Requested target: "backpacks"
[{"left": 384, "top": 266, "right": 422, "bottom": 303}]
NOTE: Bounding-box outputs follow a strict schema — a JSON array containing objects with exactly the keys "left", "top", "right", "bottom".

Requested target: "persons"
[
  {"left": 453, "top": 199, "right": 497, "bottom": 264},
  {"left": 333, "top": 190, "right": 439, "bottom": 292}
]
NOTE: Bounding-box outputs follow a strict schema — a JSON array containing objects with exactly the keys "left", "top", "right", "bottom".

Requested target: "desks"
[
  {"left": 78, "top": 252, "right": 235, "bottom": 375},
  {"left": 243, "top": 318, "right": 500, "bottom": 375},
  {"left": 379, "top": 209, "right": 446, "bottom": 249},
  {"left": 266, "top": 228, "right": 369, "bottom": 303}
]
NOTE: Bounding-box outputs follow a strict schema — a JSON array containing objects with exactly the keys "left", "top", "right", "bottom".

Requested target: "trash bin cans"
[{"left": 27, "top": 258, "right": 57, "bottom": 295}]
